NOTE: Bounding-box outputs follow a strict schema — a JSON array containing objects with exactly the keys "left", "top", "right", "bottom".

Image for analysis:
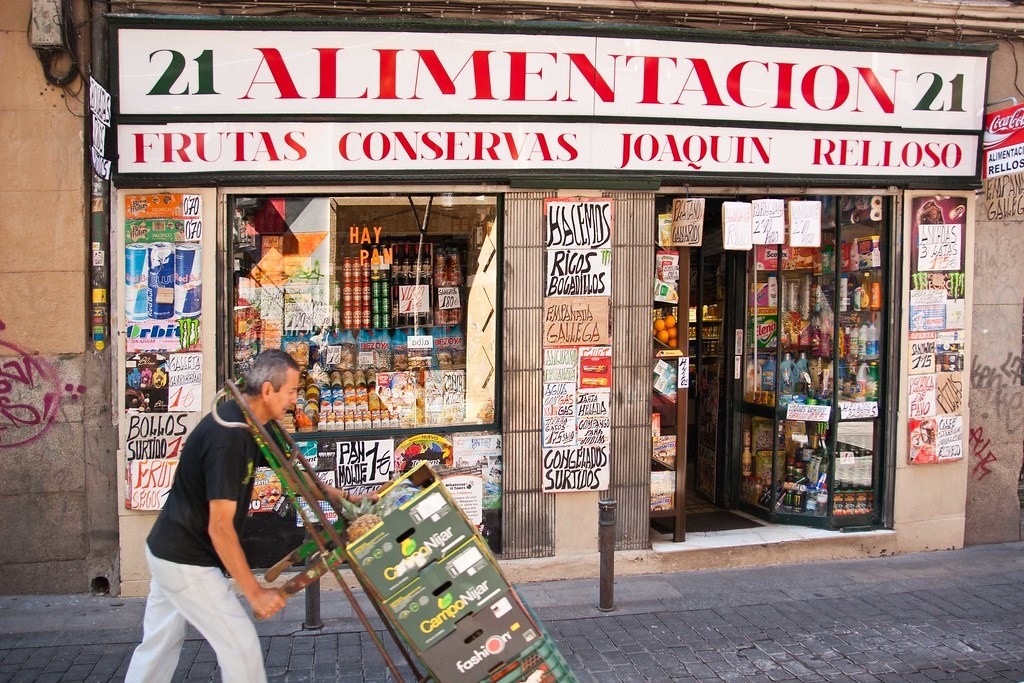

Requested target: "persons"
[{"left": 125, "top": 349, "right": 379, "bottom": 683}]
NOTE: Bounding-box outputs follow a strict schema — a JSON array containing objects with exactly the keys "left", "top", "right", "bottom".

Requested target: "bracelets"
[{"left": 343, "top": 492, "right": 349, "bottom": 499}]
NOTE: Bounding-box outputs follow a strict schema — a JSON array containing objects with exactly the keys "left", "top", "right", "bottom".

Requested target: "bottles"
[
  {"left": 412, "top": 244, "right": 422, "bottom": 285},
  {"left": 402, "top": 244, "right": 413, "bottom": 285},
  {"left": 422, "top": 243, "right": 431, "bottom": 284},
  {"left": 859, "top": 271, "right": 872, "bottom": 311},
  {"left": 390, "top": 244, "right": 402, "bottom": 286},
  {"left": 871, "top": 282, "right": 882, "bottom": 311},
  {"left": 741, "top": 446, "right": 753, "bottom": 477},
  {"left": 850, "top": 323, "right": 862, "bottom": 354},
  {"left": 867, "top": 362, "right": 879, "bottom": 402},
  {"left": 866, "top": 323, "right": 879, "bottom": 354},
  {"left": 393, "top": 286, "right": 427, "bottom": 326},
  {"left": 782, "top": 434, "right": 828, "bottom": 516},
  {"left": 859, "top": 322, "right": 870, "bottom": 356}
]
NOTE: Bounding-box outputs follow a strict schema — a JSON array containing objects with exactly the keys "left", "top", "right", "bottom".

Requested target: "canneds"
[
  {"left": 783, "top": 491, "right": 803, "bottom": 510},
  {"left": 342, "top": 256, "right": 391, "bottom": 328},
  {"left": 305, "top": 368, "right": 321, "bottom": 425}
]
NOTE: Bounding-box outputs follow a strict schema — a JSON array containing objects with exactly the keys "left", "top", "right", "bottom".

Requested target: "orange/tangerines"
[{"left": 653, "top": 315, "right": 678, "bottom": 350}]
